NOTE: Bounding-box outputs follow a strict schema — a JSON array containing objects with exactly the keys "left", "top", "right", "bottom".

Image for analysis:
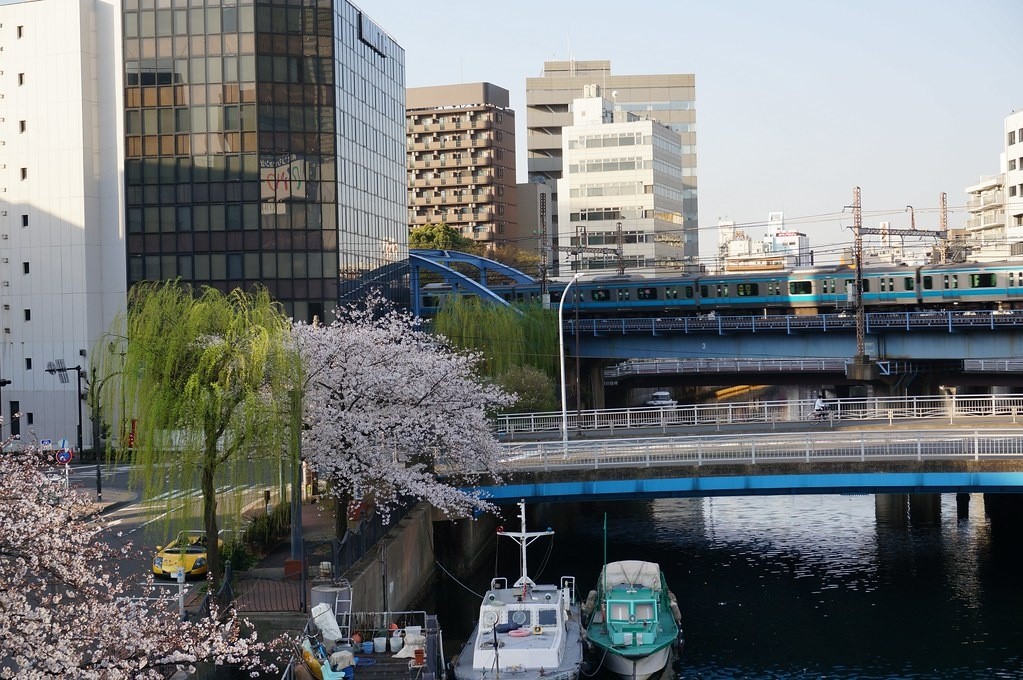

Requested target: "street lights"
[{"left": 559, "top": 272, "right": 586, "bottom": 460}]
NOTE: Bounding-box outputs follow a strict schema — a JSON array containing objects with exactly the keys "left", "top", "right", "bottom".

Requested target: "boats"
[
  {"left": 646, "top": 391, "right": 678, "bottom": 412},
  {"left": 280, "top": 590, "right": 447, "bottom": 679},
  {"left": 584, "top": 512, "right": 687, "bottom": 680},
  {"left": 451, "top": 497, "right": 588, "bottom": 680}
]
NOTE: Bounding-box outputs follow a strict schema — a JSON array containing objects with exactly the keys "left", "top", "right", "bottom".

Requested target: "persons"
[
  {"left": 707, "top": 310, "right": 716, "bottom": 320},
  {"left": 814, "top": 395, "right": 828, "bottom": 421},
  {"left": 839, "top": 310, "right": 847, "bottom": 320}
]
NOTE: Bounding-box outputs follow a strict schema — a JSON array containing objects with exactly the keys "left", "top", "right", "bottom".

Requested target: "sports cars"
[{"left": 152, "top": 529, "right": 223, "bottom": 580}]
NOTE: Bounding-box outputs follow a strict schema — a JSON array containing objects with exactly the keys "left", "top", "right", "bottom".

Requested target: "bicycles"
[{"left": 806, "top": 404, "right": 841, "bottom": 426}]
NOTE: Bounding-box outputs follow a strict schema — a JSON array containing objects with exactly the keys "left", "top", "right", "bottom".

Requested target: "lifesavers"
[
  {"left": 628, "top": 614, "right": 638, "bottom": 625},
  {"left": 507, "top": 628, "right": 531, "bottom": 638}
]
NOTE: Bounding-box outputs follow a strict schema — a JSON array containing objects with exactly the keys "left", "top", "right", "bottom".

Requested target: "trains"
[{"left": 418, "top": 260, "right": 1023, "bottom": 322}]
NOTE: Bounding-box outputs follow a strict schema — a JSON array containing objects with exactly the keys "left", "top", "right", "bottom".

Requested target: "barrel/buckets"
[
  {"left": 405, "top": 625, "right": 421, "bottom": 637},
  {"left": 415, "top": 649, "right": 424, "bottom": 666},
  {"left": 362, "top": 642, "right": 373, "bottom": 654},
  {"left": 390, "top": 636, "right": 403, "bottom": 652},
  {"left": 334, "top": 665, "right": 354, "bottom": 680},
  {"left": 373, "top": 637, "right": 387, "bottom": 653}
]
